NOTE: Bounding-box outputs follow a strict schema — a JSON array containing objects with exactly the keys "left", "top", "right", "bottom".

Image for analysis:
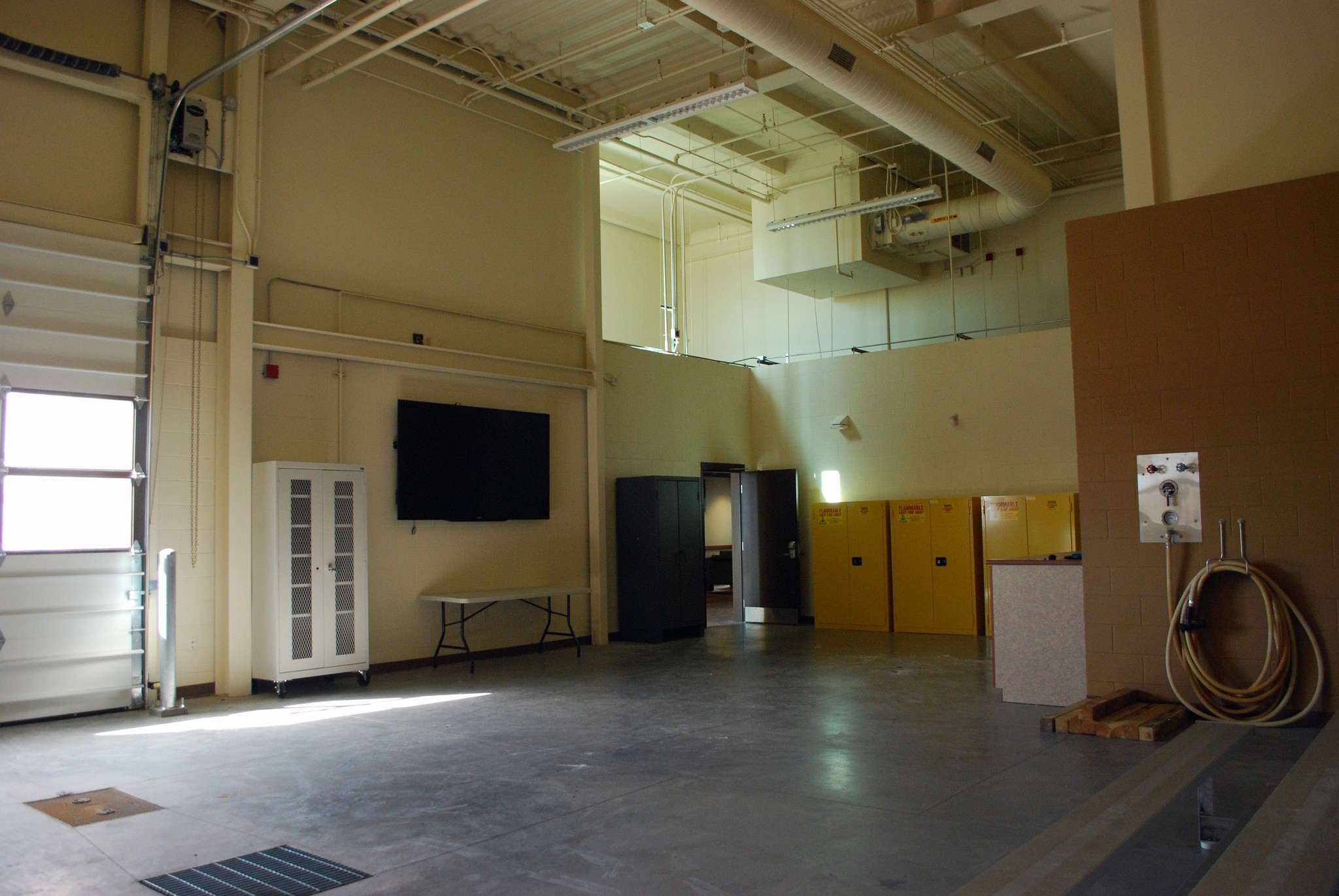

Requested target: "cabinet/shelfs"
[
  {"left": 981, "top": 493, "right": 1082, "bottom": 637},
  {"left": 252, "top": 460, "right": 369, "bottom": 699},
  {"left": 890, "top": 496, "right": 985, "bottom": 635},
  {"left": 811, "top": 500, "right": 894, "bottom": 632}
]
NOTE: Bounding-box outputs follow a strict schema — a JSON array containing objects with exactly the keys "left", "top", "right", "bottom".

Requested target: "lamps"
[
  {"left": 766, "top": 144, "right": 942, "bottom": 232},
  {"left": 830, "top": 414, "right": 849, "bottom": 430},
  {"left": 553, "top": 34, "right": 759, "bottom": 152}
]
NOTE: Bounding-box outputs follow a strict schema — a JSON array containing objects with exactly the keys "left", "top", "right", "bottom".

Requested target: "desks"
[{"left": 420, "top": 588, "right": 591, "bottom": 674}]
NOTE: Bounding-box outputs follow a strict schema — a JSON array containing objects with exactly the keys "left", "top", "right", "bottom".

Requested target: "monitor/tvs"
[{"left": 394, "top": 398, "right": 552, "bottom": 523}]
